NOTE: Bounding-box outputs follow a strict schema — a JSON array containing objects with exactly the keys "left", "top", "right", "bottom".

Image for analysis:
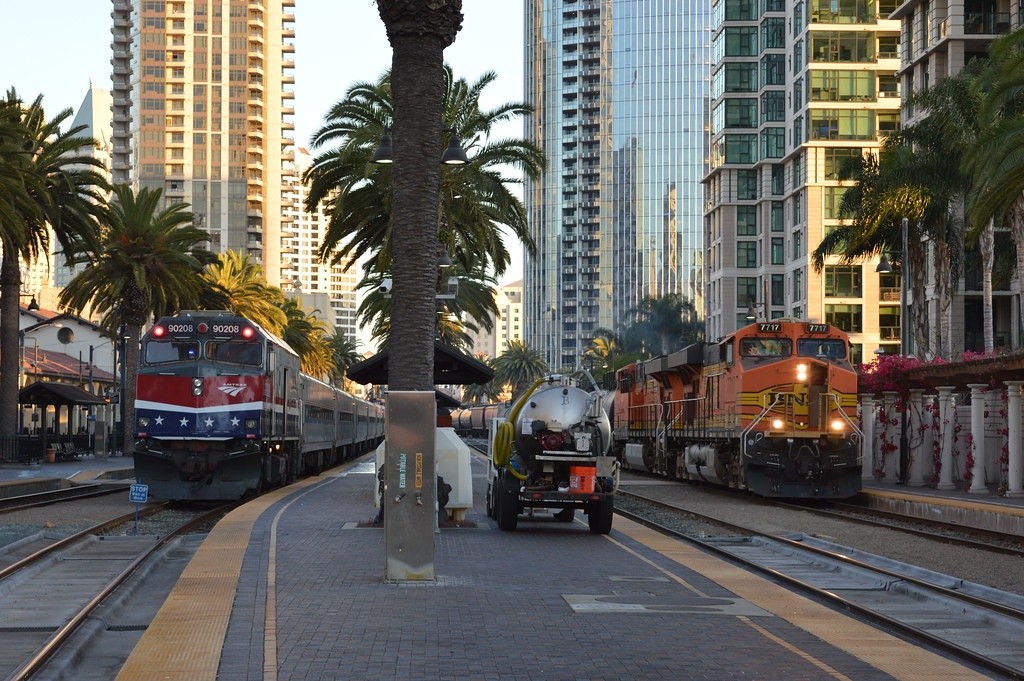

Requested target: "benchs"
[{"left": 51, "top": 442, "right": 85, "bottom": 459}]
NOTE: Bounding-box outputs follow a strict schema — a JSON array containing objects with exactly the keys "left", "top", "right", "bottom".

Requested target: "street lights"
[
  {"left": 87, "top": 324, "right": 130, "bottom": 455},
  {"left": 746, "top": 278, "right": 771, "bottom": 324},
  {"left": 874, "top": 219, "right": 911, "bottom": 486}
]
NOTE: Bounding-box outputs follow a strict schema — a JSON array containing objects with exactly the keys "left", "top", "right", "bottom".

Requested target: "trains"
[
  {"left": 132, "top": 309, "right": 385, "bottom": 502},
  {"left": 450, "top": 317, "right": 864, "bottom": 506}
]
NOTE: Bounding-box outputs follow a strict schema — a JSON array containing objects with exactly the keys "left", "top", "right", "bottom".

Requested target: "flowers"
[{"left": 858, "top": 350, "right": 1008, "bottom": 497}]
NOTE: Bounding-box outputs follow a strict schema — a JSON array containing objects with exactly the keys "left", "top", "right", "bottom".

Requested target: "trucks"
[{"left": 485, "top": 370, "right": 621, "bottom": 532}]
